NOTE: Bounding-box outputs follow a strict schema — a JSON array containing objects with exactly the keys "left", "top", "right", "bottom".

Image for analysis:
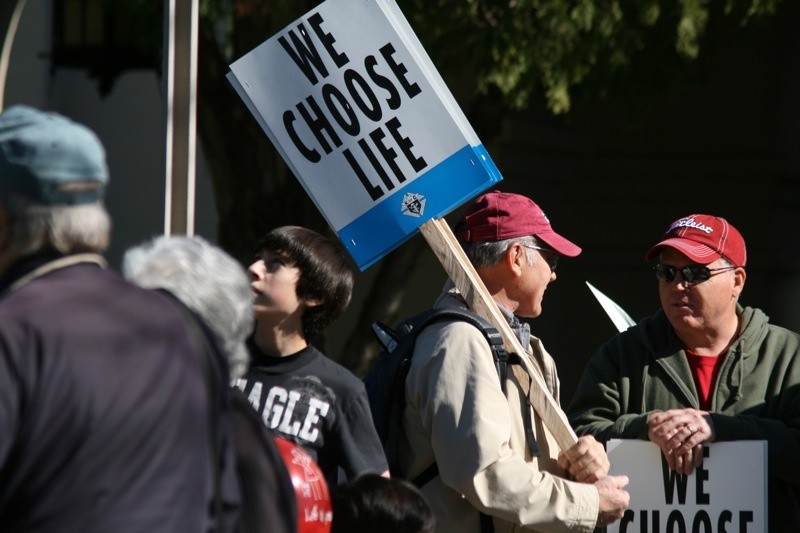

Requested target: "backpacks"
[{"left": 361, "top": 303, "right": 507, "bottom": 488}]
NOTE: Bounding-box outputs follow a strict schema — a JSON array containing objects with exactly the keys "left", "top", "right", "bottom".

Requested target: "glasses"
[
  {"left": 655, "top": 264, "right": 739, "bottom": 284},
  {"left": 498, "top": 241, "right": 560, "bottom": 273}
]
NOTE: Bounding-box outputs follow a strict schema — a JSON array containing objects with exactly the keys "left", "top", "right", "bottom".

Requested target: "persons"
[
  {"left": 568, "top": 214, "right": 800, "bottom": 533},
  {"left": 0, "top": 105, "right": 437, "bottom": 533},
  {"left": 398, "top": 189, "right": 631, "bottom": 533}
]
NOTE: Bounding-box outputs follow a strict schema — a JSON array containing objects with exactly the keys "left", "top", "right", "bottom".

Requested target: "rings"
[{"left": 687, "top": 424, "right": 694, "bottom": 435}]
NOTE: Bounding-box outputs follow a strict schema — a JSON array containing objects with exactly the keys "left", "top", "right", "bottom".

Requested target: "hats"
[
  {"left": 0, "top": 105, "right": 110, "bottom": 209},
  {"left": 645, "top": 214, "right": 746, "bottom": 272},
  {"left": 458, "top": 189, "right": 582, "bottom": 257}
]
